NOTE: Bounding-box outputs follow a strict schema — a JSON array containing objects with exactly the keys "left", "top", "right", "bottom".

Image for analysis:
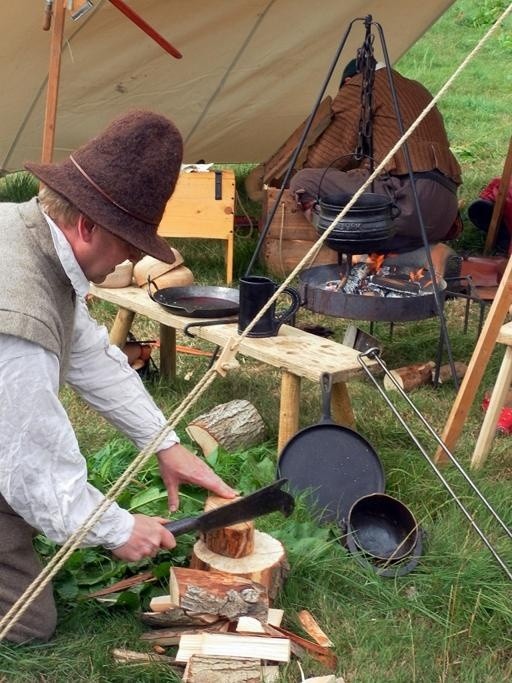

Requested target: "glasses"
[{"left": 127, "top": 247, "right": 145, "bottom": 263}]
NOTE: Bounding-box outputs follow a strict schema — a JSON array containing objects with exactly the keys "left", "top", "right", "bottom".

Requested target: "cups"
[{"left": 238, "top": 275, "right": 299, "bottom": 336}]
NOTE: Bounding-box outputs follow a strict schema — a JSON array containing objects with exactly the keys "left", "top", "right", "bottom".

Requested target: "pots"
[
  {"left": 275, "top": 371, "right": 385, "bottom": 533},
  {"left": 145, "top": 279, "right": 239, "bottom": 319},
  {"left": 311, "top": 193, "right": 398, "bottom": 253}
]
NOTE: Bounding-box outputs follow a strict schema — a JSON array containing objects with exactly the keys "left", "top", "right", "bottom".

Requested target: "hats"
[{"left": 23, "top": 109, "right": 183, "bottom": 264}]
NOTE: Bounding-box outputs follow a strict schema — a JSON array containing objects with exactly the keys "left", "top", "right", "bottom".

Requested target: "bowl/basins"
[
  {"left": 95, "top": 248, "right": 194, "bottom": 292},
  {"left": 348, "top": 491, "right": 418, "bottom": 560}
]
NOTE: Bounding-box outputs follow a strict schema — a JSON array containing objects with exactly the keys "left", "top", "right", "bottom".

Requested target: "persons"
[
  {"left": 290, "top": 56, "right": 464, "bottom": 254},
  {"left": 0, "top": 107, "right": 241, "bottom": 646}
]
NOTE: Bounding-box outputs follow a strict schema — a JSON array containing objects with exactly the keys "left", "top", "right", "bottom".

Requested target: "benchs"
[{"left": 88, "top": 281, "right": 386, "bottom": 466}]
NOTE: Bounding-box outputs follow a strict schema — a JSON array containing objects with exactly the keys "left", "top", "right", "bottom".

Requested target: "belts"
[{"left": 401, "top": 171, "right": 458, "bottom": 195}]
